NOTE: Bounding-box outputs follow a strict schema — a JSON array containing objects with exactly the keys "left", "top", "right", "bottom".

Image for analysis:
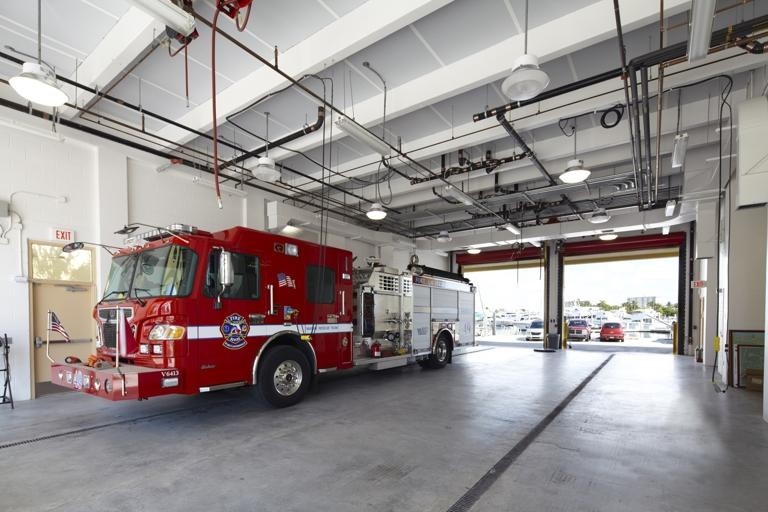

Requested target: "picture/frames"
[{"left": 728, "top": 329, "right": 764, "bottom": 386}]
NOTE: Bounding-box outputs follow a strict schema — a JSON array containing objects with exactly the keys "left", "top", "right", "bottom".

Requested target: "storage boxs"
[{"left": 743, "top": 368, "right": 763, "bottom": 392}]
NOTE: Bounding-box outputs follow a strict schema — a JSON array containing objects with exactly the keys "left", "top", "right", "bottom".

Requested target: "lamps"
[
  {"left": 252, "top": 111, "right": 452, "bottom": 244},
  {"left": 499, "top": 1, "right": 611, "bottom": 224},
  {"left": 8, "top": 1, "right": 70, "bottom": 106},
  {"left": 664, "top": 88, "right": 690, "bottom": 218}
]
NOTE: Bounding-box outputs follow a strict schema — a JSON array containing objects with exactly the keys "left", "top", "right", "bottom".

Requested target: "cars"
[
  {"left": 567, "top": 319, "right": 591, "bottom": 341},
  {"left": 525, "top": 320, "right": 544, "bottom": 341},
  {"left": 600, "top": 321, "right": 624, "bottom": 343}
]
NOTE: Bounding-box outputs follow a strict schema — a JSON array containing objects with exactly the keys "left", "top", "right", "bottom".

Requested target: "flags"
[
  {"left": 119, "top": 310, "right": 140, "bottom": 358},
  {"left": 51, "top": 311, "right": 71, "bottom": 344},
  {"left": 278, "top": 273, "right": 296, "bottom": 288}
]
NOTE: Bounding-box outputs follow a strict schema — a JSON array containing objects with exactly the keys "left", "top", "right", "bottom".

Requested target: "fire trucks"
[{"left": 46, "top": 221, "right": 477, "bottom": 407}]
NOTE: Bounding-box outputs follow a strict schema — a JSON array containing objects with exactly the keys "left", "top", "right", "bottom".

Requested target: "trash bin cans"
[{"left": 547, "top": 334, "right": 561, "bottom": 350}]
